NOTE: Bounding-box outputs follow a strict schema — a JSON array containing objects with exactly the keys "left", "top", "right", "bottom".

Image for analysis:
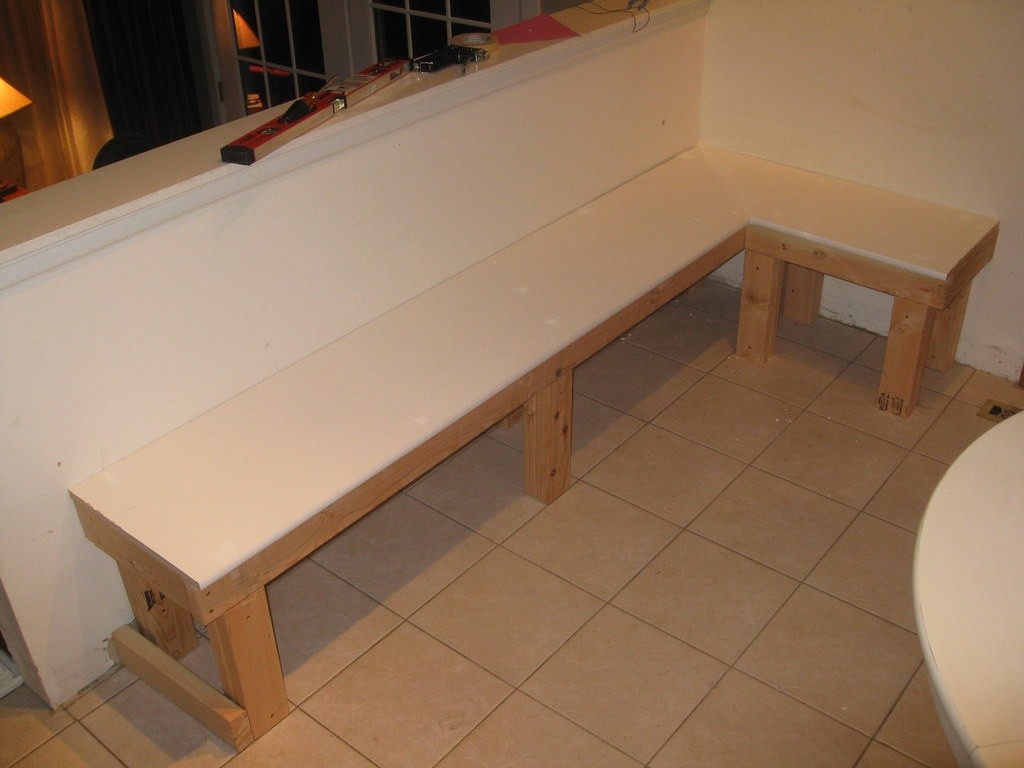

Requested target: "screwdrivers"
[{"left": 278, "top": 73, "right": 339, "bottom": 125}]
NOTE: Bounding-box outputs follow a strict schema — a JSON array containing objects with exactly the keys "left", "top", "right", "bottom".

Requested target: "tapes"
[{"left": 452, "top": 32, "right": 500, "bottom": 53}]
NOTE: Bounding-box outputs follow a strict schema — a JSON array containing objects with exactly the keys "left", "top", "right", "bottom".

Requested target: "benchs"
[{"left": 67, "top": 146, "right": 1002, "bottom": 755}]
